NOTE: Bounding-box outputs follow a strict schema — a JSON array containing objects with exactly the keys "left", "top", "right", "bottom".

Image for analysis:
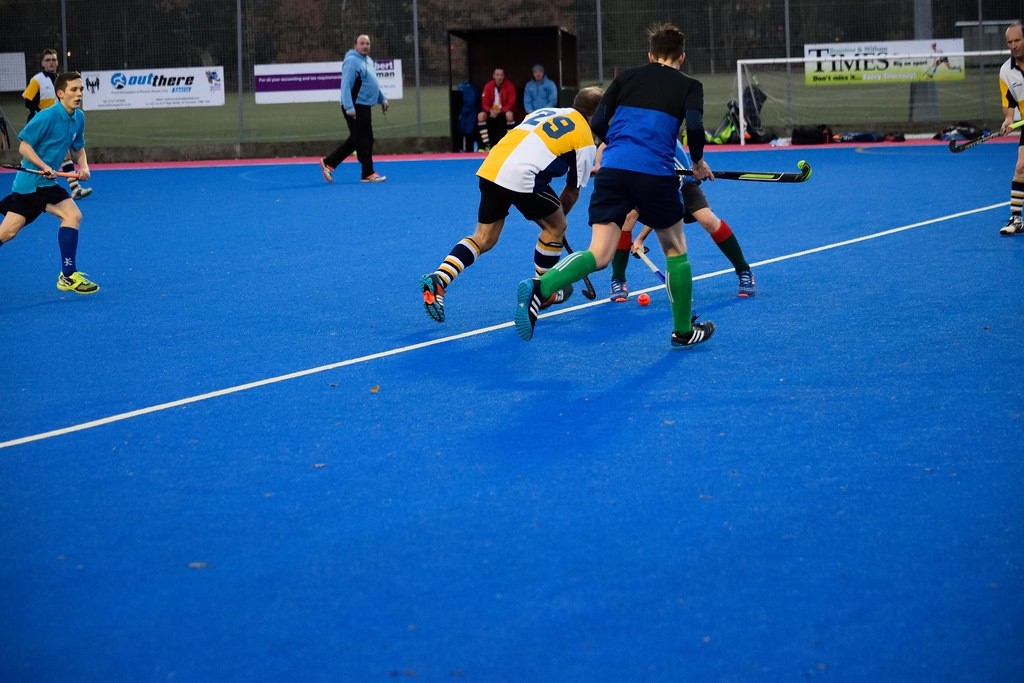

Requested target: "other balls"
[{"left": 637, "top": 292, "right": 651, "bottom": 307}]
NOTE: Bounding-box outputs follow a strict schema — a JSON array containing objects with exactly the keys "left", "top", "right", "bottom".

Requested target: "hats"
[{"left": 531, "top": 64, "right": 544, "bottom": 71}]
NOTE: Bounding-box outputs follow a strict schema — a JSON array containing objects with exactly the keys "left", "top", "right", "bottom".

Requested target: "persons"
[
  {"left": 420, "top": 87, "right": 605, "bottom": 324},
  {"left": 515, "top": 26, "right": 756, "bottom": 347},
  {"left": 523, "top": 64, "right": 557, "bottom": 114},
  {"left": 478, "top": 66, "right": 517, "bottom": 153},
  {"left": 927, "top": 42, "right": 962, "bottom": 78},
  {"left": 0, "top": 72, "right": 101, "bottom": 295},
  {"left": 999, "top": 22, "right": 1024, "bottom": 234},
  {"left": 321, "top": 34, "right": 390, "bottom": 184},
  {"left": 22, "top": 49, "right": 93, "bottom": 200}
]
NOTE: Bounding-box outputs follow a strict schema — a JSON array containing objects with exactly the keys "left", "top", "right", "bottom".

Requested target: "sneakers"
[
  {"left": 610, "top": 276, "right": 629, "bottom": 302},
  {"left": 477, "top": 144, "right": 492, "bottom": 154},
  {"left": 360, "top": 172, "right": 387, "bottom": 183},
  {"left": 513, "top": 277, "right": 548, "bottom": 342},
  {"left": 419, "top": 272, "right": 446, "bottom": 323},
  {"left": 71, "top": 186, "right": 93, "bottom": 201},
  {"left": 670, "top": 312, "right": 716, "bottom": 347},
  {"left": 735, "top": 269, "right": 755, "bottom": 297},
  {"left": 539, "top": 284, "right": 574, "bottom": 310},
  {"left": 999, "top": 215, "right": 1024, "bottom": 236},
  {"left": 320, "top": 158, "right": 334, "bottom": 182},
  {"left": 56, "top": 270, "right": 100, "bottom": 294}
]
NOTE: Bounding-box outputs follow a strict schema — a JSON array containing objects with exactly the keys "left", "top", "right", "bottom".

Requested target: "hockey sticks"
[
  {"left": 948, "top": 119, "right": 1024, "bottom": 154},
  {"left": 591, "top": 160, "right": 813, "bottom": 183},
  {"left": 561, "top": 237, "right": 597, "bottom": 301},
  {"left": 630, "top": 242, "right": 703, "bottom": 324},
  {"left": 0, "top": 159, "right": 80, "bottom": 179}
]
